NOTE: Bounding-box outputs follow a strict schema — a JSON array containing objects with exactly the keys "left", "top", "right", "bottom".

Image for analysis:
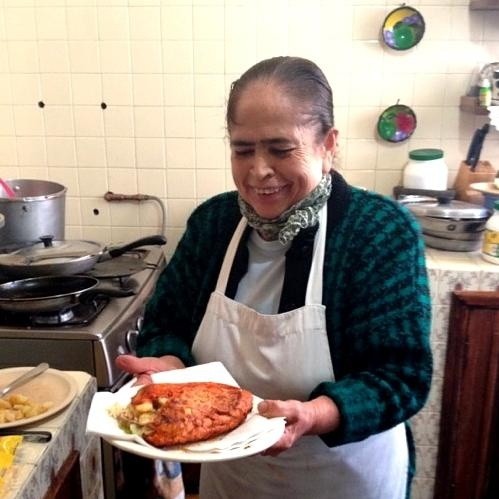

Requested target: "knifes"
[{"left": 464, "top": 123, "right": 490, "bottom": 170}]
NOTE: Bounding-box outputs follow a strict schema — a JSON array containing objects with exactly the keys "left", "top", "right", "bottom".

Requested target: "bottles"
[
  {"left": 483, "top": 200, "right": 499, "bottom": 264},
  {"left": 478, "top": 73, "right": 493, "bottom": 108}
]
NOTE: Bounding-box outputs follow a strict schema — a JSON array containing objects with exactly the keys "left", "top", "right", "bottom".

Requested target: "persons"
[{"left": 113, "top": 57, "right": 434, "bottom": 499}]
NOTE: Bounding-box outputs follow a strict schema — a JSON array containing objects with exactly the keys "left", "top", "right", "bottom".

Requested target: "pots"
[
  {"left": 402, "top": 196, "right": 491, "bottom": 254},
  {"left": 0, "top": 178, "right": 167, "bottom": 316}
]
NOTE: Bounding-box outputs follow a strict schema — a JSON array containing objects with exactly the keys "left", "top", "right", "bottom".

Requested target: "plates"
[
  {"left": 0, "top": 366, "right": 77, "bottom": 430},
  {"left": 377, "top": 104, "right": 417, "bottom": 143},
  {"left": 96, "top": 416, "right": 288, "bottom": 465},
  {"left": 382, "top": 5, "right": 426, "bottom": 51},
  {"left": 469, "top": 181, "right": 499, "bottom": 194}
]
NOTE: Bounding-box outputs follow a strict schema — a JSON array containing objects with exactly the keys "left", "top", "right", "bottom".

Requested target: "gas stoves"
[{"left": 0, "top": 245, "right": 167, "bottom": 388}]
